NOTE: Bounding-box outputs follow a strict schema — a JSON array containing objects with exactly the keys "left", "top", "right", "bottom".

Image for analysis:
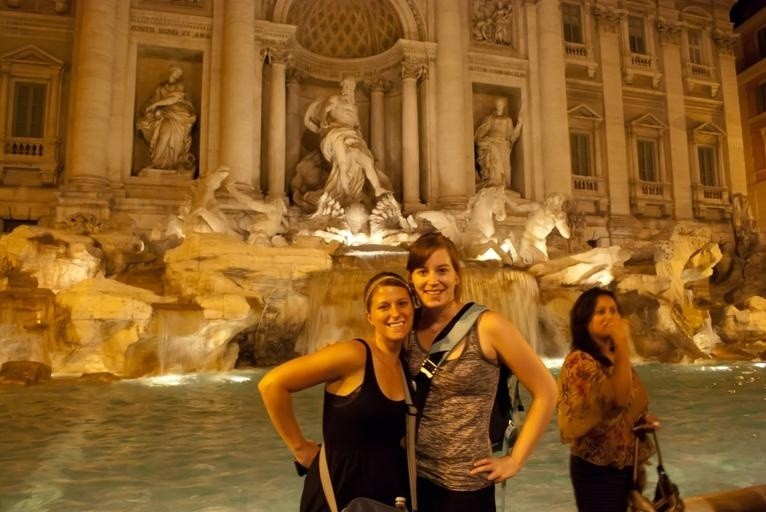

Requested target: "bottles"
[{"left": 394, "top": 496, "right": 408, "bottom": 512}]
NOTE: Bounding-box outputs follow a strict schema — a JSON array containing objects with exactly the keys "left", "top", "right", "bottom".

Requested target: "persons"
[
  {"left": 141, "top": 65, "right": 185, "bottom": 171},
  {"left": 319, "top": 74, "right": 394, "bottom": 206},
  {"left": 476, "top": 96, "right": 524, "bottom": 187},
  {"left": 256, "top": 271, "right": 415, "bottom": 512},
  {"left": 316, "top": 232, "right": 559, "bottom": 512},
  {"left": 498, "top": 193, "right": 574, "bottom": 265},
  {"left": 491, "top": 1, "right": 512, "bottom": 43},
  {"left": 556, "top": 286, "right": 658, "bottom": 512}
]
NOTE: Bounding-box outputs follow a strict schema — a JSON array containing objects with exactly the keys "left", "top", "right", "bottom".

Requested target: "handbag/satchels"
[
  {"left": 411, "top": 302, "right": 525, "bottom": 453},
  {"left": 626, "top": 430, "right": 685, "bottom": 512},
  {"left": 341, "top": 497, "right": 409, "bottom": 511}
]
